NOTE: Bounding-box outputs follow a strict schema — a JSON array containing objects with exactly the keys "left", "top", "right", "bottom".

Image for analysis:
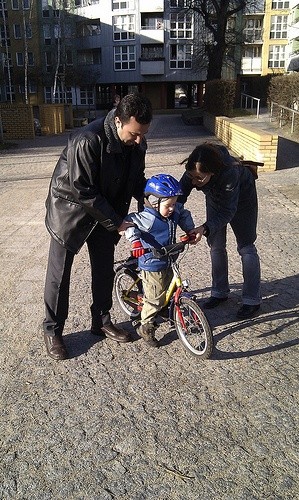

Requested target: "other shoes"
[
  {"left": 203, "top": 296, "right": 228, "bottom": 309},
  {"left": 236, "top": 304, "right": 261, "bottom": 319}
]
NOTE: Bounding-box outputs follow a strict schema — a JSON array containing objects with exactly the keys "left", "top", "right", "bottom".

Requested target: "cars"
[{"left": 179, "top": 96, "right": 187, "bottom": 107}]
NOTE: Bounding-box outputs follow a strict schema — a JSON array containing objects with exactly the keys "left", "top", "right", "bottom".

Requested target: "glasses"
[{"left": 186, "top": 173, "right": 207, "bottom": 184}]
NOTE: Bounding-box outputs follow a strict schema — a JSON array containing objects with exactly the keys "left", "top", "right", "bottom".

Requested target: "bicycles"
[{"left": 113, "top": 233, "right": 214, "bottom": 360}]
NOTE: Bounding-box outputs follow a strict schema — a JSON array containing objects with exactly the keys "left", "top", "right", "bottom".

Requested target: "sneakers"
[{"left": 136, "top": 323, "right": 159, "bottom": 347}]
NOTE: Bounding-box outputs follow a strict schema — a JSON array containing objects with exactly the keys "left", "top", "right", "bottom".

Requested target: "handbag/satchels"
[{"left": 240, "top": 160, "right": 264, "bottom": 179}]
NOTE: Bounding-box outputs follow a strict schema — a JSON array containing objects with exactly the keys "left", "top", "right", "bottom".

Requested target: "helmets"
[{"left": 143, "top": 173, "right": 183, "bottom": 198}]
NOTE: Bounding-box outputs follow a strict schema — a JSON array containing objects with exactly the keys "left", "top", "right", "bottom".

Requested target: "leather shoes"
[
  {"left": 44, "top": 334, "right": 67, "bottom": 360},
  {"left": 91, "top": 322, "right": 131, "bottom": 343}
]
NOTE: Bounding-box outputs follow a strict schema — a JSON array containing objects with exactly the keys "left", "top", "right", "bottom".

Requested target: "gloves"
[
  {"left": 187, "top": 233, "right": 196, "bottom": 239},
  {"left": 132, "top": 240, "right": 145, "bottom": 258}
]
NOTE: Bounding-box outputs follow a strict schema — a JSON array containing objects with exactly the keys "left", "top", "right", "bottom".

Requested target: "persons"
[
  {"left": 111, "top": 95, "right": 120, "bottom": 108},
  {"left": 176, "top": 143, "right": 261, "bottom": 319},
  {"left": 123, "top": 174, "right": 197, "bottom": 346},
  {"left": 43, "top": 93, "right": 153, "bottom": 360}
]
